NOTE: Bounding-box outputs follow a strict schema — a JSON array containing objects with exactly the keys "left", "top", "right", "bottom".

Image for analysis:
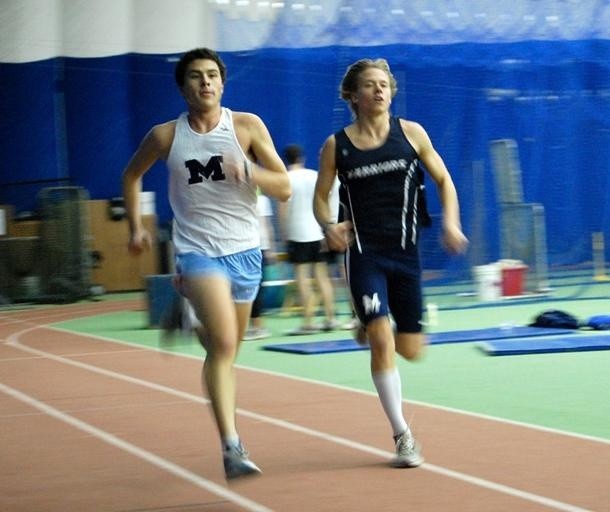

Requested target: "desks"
[{"left": 0, "top": 199, "right": 158, "bottom": 294}]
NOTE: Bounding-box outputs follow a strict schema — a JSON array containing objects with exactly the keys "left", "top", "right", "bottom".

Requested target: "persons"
[
  {"left": 125, "top": 46, "right": 291, "bottom": 478},
  {"left": 237, "top": 194, "right": 277, "bottom": 339},
  {"left": 311, "top": 56, "right": 469, "bottom": 469},
  {"left": 279, "top": 145, "right": 343, "bottom": 330}
]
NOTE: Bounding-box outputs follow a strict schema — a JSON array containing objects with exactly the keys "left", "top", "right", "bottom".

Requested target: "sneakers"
[
  {"left": 356, "top": 321, "right": 367, "bottom": 346},
  {"left": 244, "top": 328, "right": 268, "bottom": 340},
  {"left": 394, "top": 439, "right": 423, "bottom": 468},
  {"left": 223, "top": 455, "right": 262, "bottom": 481},
  {"left": 284, "top": 319, "right": 360, "bottom": 336}
]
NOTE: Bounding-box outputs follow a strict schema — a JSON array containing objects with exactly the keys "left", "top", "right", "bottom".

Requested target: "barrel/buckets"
[
  {"left": 500, "top": 259, "right": 528, "bottom": 296},
  {"left": 471, "top": 265, "right": 501, "bottom": 300},
  {"left": 139, "top": 191, "right": 158, "bottom": 215},
  {"left": 18, "top": 276, "right": 41, "bottom": 295},
  {"left": 143, "top": 273, "right": 181, "bottom": 326}
]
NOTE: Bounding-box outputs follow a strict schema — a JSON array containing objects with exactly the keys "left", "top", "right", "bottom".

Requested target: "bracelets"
[{"left": 321, "top": 222, "right": 335, "bottom": 235}]
